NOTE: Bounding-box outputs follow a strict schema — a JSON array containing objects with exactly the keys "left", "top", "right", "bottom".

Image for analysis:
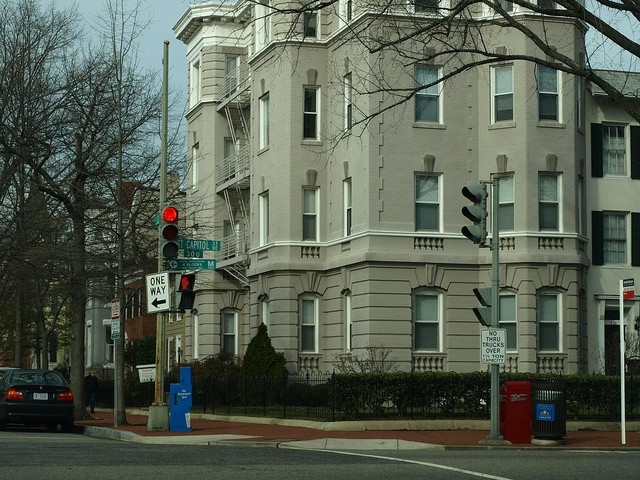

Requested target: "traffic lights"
[
  {"left": 159, "top": 205, "right": 185, "bottom": 259},
  {"left": 472, "top": 286, "right": 495, "bottom": 327},
  {"left": 175, "top": 292, "right": 198, "bottom": 315},
  {"left": 173, "top": 273, "right": 195, "bottom": 291},
  {"left": 461, "top": 181, "right": 489, "bottom": 245}
]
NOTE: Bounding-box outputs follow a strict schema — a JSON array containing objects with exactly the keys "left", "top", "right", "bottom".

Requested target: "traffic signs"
[
  {"left": 176, "top": 238, "right": 221, "bottom": 259},
  {"left": 145, "top": 273, "right": 175, "bottom": 315},
  {"left": 481, "top": 328, "right": 506, "bottom": 364},
  {"left": 623, "top": 286, "right": 635, "bottom": 291},
  {"left": 623, "top": 291, "right": 634, "bottom": 300},
  {"left": 160, "top": 258, "right": 217, "bottom": 274},
  {"left": 623, "top": 278, "right": 634, "bottom": 286}
]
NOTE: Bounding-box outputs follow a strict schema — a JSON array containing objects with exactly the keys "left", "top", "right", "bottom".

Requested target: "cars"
[{"left": 0, "top": 370, "right": 73, "bottom": 434}]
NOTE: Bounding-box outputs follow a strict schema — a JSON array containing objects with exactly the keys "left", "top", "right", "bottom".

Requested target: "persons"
[
  {"left": 54, "top": 362, "right": 66, "bottom": 377},
  {"left": 85, "top": 370, "right": 99, "bottom": 414},
  {"left": 62, "top": 359, "right": 72, "bottom": 388}
]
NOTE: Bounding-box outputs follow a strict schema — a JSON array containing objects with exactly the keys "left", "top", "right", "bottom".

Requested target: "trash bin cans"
[
  {"left": 531, "top": 389, "right": 567, "bottom": 440},
  {"left": 500, "top": 380, "right": 531, "bottom": 444}
]
